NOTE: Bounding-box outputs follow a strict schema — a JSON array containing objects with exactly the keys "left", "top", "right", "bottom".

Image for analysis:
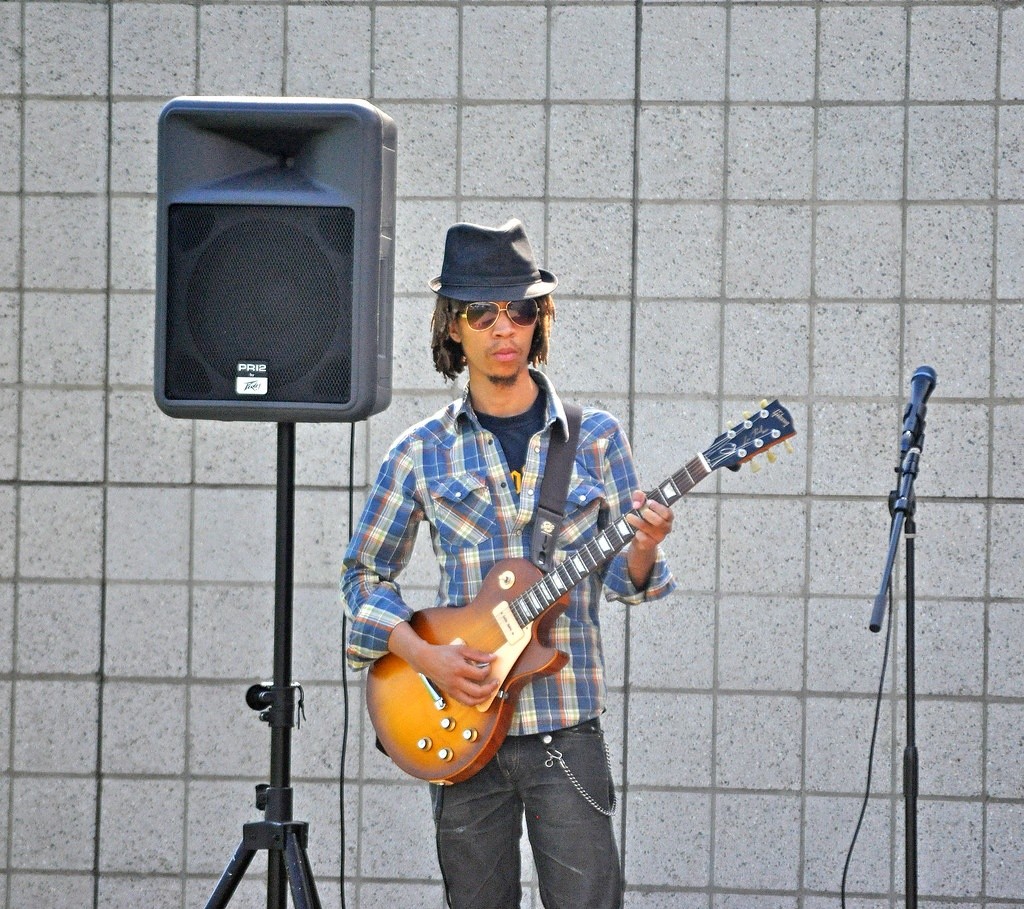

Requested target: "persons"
[{"left": 334, "top": 216, "right": 676, "bottom": 909}]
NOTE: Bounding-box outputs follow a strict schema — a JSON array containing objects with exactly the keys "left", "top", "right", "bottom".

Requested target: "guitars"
[{"left": 366, "top": 399, "right": 799, "bottom": 787}]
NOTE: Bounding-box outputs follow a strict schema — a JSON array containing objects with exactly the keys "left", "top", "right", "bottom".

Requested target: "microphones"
[{"left": 900, "top": 366, "right": 936, "bottom": 453}]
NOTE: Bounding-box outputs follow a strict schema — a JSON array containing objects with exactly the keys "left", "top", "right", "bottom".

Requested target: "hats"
[{"left": 426, "top": 217, "right": 560, "bottom": 302}]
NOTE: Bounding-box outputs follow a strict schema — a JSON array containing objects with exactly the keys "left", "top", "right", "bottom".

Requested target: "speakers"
[{"left": 155, "top": 96, "right": 399, "bottom": 423}]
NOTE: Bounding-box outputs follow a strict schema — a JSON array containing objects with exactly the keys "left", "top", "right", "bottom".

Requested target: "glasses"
[{"left": 458, "top": 301, "right": 540, "bottom": 331}]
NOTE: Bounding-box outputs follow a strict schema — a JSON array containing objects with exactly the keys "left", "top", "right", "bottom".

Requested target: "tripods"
[{"left": 203, "top": 422, "right": 324, "bottom": 909}]
